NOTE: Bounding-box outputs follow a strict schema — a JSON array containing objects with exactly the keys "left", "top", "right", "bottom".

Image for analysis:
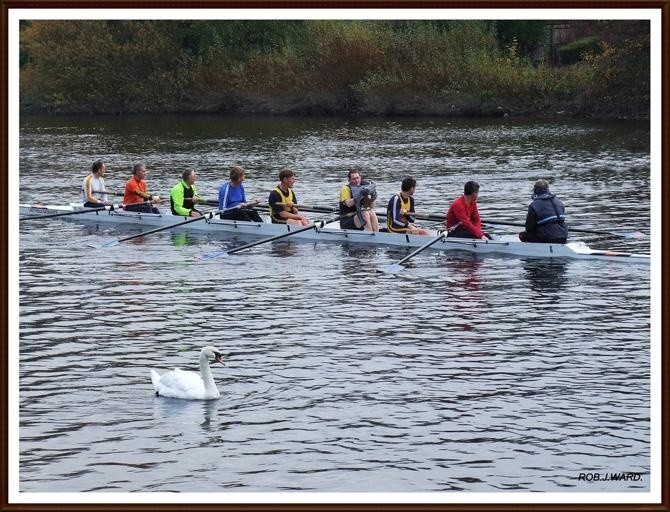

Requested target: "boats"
[{"left": 19, "top": 203, "right": 651, "bottom": 263}]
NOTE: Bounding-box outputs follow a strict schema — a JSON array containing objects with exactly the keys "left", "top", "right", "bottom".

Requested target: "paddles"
[
  {"left": 404, "top": 212, "right": 619, "bottom": 239},
  {"left": 376, "top": 222, "right": 462, "bottom": 274},
  {"left": 88, "top": 201, "right": 258, "bottom": 250},
  {"left": 198, "top": 210, "right": 359, "bottom": 261}
]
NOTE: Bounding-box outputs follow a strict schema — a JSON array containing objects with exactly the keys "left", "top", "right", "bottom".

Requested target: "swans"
[{"left": 148, "top": 344, "right": 228, "bottom": 401}]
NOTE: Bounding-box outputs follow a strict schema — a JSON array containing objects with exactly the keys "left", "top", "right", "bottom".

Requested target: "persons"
[
  {"left": 446, "top": 181, "right": 489, "bottom": 240},
  {"left": 122, "top": 163, "right": 160, "bottom": 214},
  {"left": 267, "top": 169, "right": 309, "bottom": 226},
  {"left": 82, "top": 160, "right": 109, "bottom": 207},
  {"left": 217, "top": 167, "right": 263, "bottom": 222},
  {"left": 519, "top": 180, "right": 568, "bottom": 243},
  {"left": 169, "top": 169, "right": 203, "bottom": 217},
  {"left": 386, "top": 178, "right": 428, "bottom": 235},
  {"left": 339, "top": 170, "right": 380, "bottom": 232}
]
